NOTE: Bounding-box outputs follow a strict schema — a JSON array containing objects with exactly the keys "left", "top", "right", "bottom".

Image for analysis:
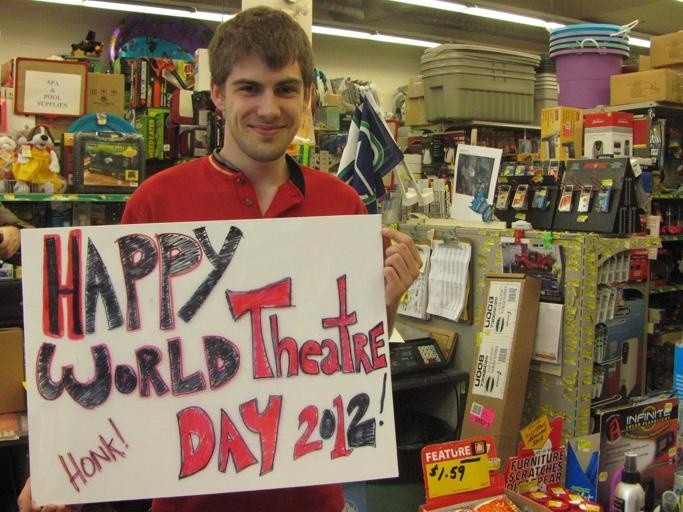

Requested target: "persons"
[
  {"left": 459, "top": 154, "right": 476, "bottom": 196},
  {"left": 471, "top": 157, "right": 491, "bottom": 198},
  {"left": 16, "top": 5, "right": 424, "bottom": 511}
]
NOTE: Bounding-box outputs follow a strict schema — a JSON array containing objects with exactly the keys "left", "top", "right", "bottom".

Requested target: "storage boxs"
[
  {"left": 35, "top": 72, "right": 125, "bottom": 140},
  {"left": 541, "top": 105, "right": 635, "bottom": 160},
  {"left": 460, "top": 272, "right": 542, "bottom": 477},
  {"left": 609, "top": 30, "right": 683, "bottom": 106},
  {"left": 1, "top": 327, "right": 26, "bottom": 417},
  {"left": 405, "top": 76, "right": 435, "bottom": 127}
]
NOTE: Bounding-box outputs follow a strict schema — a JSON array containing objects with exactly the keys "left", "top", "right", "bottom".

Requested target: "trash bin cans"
[{"left": 364, "top": 412, "right": 454, "bottom": 512}]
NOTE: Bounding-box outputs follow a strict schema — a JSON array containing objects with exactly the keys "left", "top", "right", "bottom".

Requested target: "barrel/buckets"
[{"left": 547, "top": 20, "right": 640, "bottom": 106}]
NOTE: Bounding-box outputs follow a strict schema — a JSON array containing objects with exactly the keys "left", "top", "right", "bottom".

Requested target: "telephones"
[{"left": 387, "top": 338, "right": 447, "bottom": 379}]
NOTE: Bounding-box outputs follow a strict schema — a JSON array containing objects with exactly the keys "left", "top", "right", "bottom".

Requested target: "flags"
[
  {"left": 332, "top": 104, "right": 396, "bottom": 214},
  {"left": 350, "top": 91, "right": 407, "bottom": 207}
]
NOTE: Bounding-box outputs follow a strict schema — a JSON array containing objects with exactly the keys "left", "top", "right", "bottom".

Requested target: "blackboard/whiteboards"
[{"left": 19, "top": 214, "right": 400, "bottom": 507}]
